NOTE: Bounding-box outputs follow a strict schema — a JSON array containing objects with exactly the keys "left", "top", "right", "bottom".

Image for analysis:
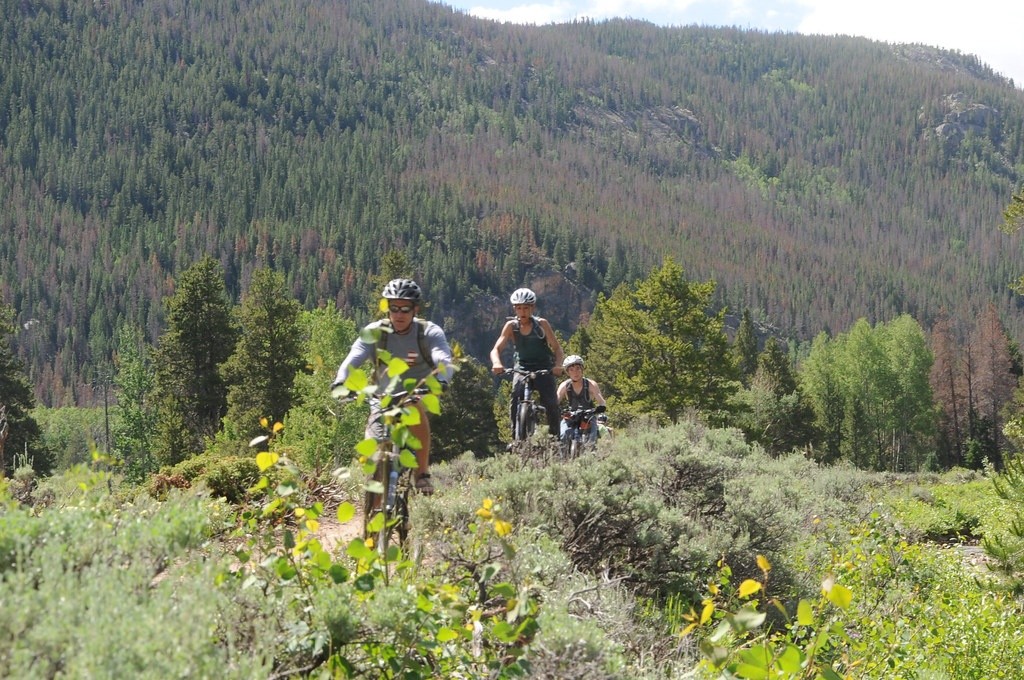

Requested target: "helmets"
[
  {"left": 383, "top": 278, "right": 423, "bottom": 300},
  {"left": 563, "top": 355, "right": 584, "bottom": 371},
  {"left": 510, "top": 288, "right": 536, "bottom": 305},
  {"left": 597, "top": 414, "right": 607, "bottom": 424}
]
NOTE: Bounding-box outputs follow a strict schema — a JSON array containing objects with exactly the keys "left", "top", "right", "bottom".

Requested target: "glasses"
[{"left": 388, "top": 305, "right": 413, "bottom": 313}]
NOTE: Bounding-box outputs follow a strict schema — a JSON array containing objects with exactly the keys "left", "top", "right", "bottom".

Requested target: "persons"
[
  {"left": 597, "top": 414, "right": 612, "bottom": 432},
  {"left": 329, "top": 278, "right": 454, "bottom": 553},
  {"left": 554, "top": 354, "right": 606, "bottom": 459},
  {"left": 489, "top": 288, "right": 563, "bottom": 453}
]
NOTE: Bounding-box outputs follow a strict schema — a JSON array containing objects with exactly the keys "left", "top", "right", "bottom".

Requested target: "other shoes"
[
  {"left": 508, "top": 441, "right": 523, "bottom": 452},
  {"left": 550, "top": 435, "right": 561, "bottom": 447}
]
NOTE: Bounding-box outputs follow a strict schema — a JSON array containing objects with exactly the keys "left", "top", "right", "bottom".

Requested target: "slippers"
[{"left": 413, "top": 472, "right": 434, "bottom": 497}]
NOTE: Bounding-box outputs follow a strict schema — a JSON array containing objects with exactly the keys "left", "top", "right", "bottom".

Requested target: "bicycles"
[
  {"left": 352, "top": 388, "right": 430, "bottom": 558},
  {"left": 559, "top": 406, "right": 597, "bottom": 460},
  {"left": 498, "top": 368, "right": 564, "bottom": 449}
]
